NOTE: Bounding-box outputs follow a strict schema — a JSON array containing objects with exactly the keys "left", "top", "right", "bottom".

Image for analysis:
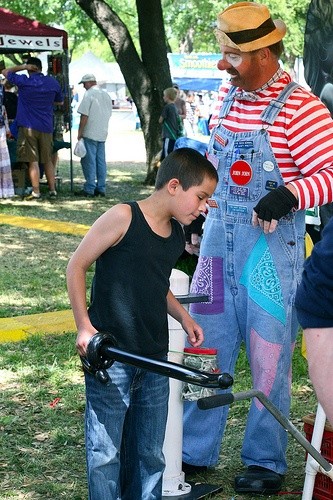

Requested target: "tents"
[{"left": 0, "top": 8, "right": 67, "bottom": 52}]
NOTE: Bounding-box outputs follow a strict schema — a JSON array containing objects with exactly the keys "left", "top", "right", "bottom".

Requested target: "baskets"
[{"left": 301, "top": 410, "right": 333, "bottom": 500}]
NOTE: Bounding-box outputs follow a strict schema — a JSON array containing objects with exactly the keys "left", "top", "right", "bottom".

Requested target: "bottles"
[{"left": 180, "top": 347, "right": 221, "bottom": 403}]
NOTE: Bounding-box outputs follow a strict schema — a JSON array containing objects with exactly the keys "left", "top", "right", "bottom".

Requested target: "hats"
[
  {"left": 213, "top": 2, "right": 286, "bottom": 52},
  {"left": 78, "top": 73, "right": 97, "bottom": 85}
]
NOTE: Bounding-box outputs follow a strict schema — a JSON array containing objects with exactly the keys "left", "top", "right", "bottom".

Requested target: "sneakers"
[
  {"left": 49, "top": 191, "right": 57, "bottom": 200},
  {"left": 24, "top": 191, "right": 41, "bottom": 201}
]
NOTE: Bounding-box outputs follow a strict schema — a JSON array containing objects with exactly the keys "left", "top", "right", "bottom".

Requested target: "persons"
[
  {"left": 0, "top": 56, "right": 219, "bottom": 201},
  {"left": 294, "top": 214, "right": 333, "bottom": 429},
  {"left": 66, "top": 147, "right": 220, "bottom": 500},
  {"left": 182, "top": 2, "right": 333, "bottom": 496}
]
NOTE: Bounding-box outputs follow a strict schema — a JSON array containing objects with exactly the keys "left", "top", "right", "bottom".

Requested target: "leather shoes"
[
  {"left": 74, "top": 190, "right": 94, "bottom": 198},
  {"left": 94, "top": 190, "right": 105, "bottom": 197},
  {"left": 234, "top": 465, "right": 282, "bottom": 494},
  {"left": 182, "top": 461, "right": 207, "bottom": 475}
]
